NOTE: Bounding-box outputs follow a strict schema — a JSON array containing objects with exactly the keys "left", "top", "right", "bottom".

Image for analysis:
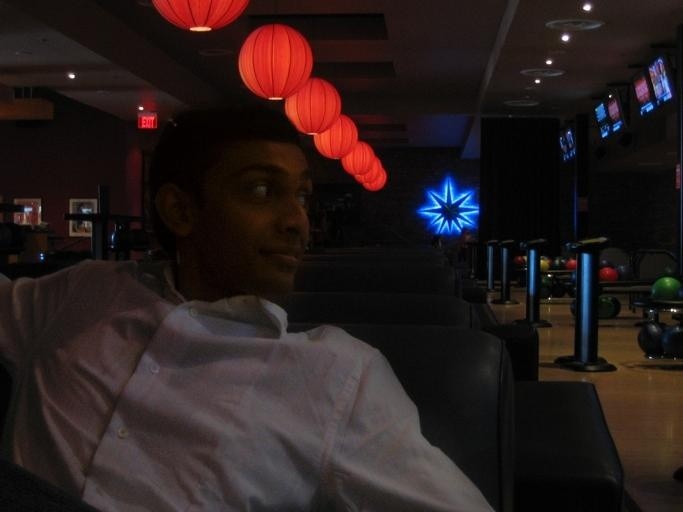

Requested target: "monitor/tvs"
[{"left": 556, "top": 54, "right": 675, "bottom": 161}]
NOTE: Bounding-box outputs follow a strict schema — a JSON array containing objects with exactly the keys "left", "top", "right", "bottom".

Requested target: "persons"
[{"left": 0, "top": 105, "right": 491, "bottom": 512}]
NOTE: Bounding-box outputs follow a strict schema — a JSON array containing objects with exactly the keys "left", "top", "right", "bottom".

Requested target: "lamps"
[
  {"left": 237, "top": 22, "right": 315, "bottom": 101},
  {"left": 362, "top": 169, "right": 388, "bottom": 192},
  {"left": 340, "top": 139, "right": 374, "bottom": 177},
  {"left": 312, "top": 113, "right": 359, "bottom": 161},
  {"left": 283, "top": 74, "right": 343, "bottom": 136},
  {"left": 352, "top": 156, "right": 382, "bottom": 185}
]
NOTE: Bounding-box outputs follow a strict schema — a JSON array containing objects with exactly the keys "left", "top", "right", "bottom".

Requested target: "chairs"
[{"left": 278, "top": 248, "right": 628, "bottom": 512}]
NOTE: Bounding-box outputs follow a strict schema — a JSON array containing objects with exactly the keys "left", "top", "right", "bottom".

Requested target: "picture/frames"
[
  {"left": 8, "top": 195, "right": 44, "bottom": 227},
  {"left": 69, "top": 198, "right": 97, "bottom": 238}
]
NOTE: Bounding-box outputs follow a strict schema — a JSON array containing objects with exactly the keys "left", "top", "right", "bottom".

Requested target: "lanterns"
[{"left": 238, "top": 23, "right": 387, "bottom": 192}]
